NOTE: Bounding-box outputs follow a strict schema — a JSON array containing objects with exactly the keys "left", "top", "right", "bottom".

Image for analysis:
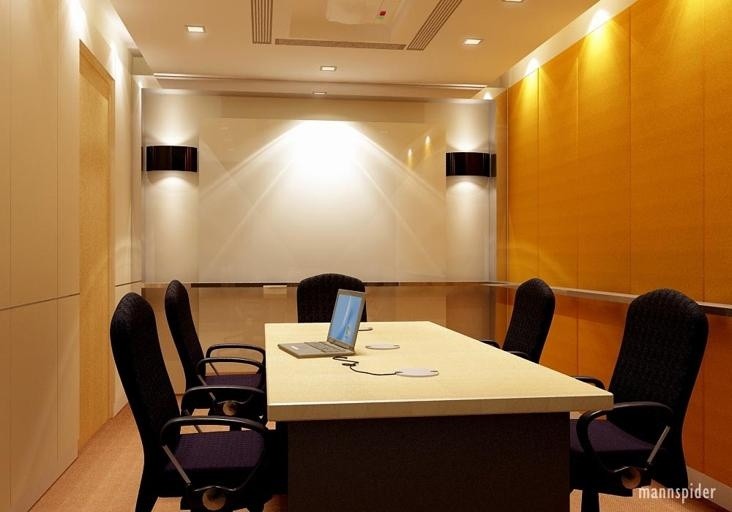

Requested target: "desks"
[{"left": 265, "top": 318, "right": 614, "bottom": 510}]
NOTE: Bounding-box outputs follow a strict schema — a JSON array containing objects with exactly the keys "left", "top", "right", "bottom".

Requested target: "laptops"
[{"left": 278, "top": 288, "right": 367, "bottom": 359}]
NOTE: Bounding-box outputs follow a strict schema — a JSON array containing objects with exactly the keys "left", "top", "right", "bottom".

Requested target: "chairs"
[
  {"left": 296, "top": 273, "right": 367, "bottom": 322},
  {"left": 480, "top": 278, "right": 710, "bottom": 512},
  {"left": 110, "top": 278, "right": 277, "bottom": 512}
]
